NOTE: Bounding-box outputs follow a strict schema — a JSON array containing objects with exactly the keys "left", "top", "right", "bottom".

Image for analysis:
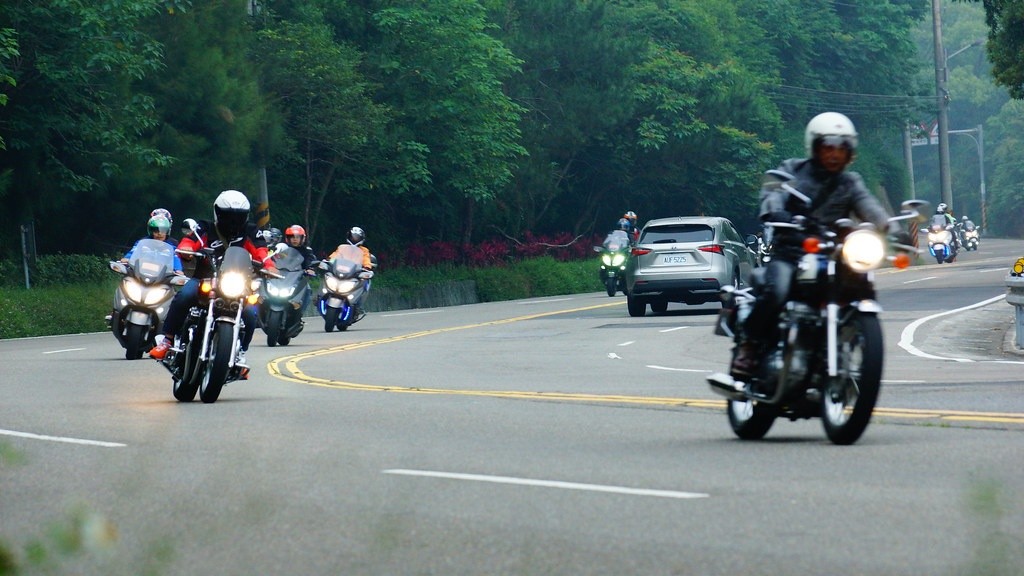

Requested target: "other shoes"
[{"left": 731, "top": 345, "right": 754, "bottom": 375}]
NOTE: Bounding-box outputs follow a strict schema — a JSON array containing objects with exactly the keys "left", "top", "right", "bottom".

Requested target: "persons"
[
  {"left": 959, "top": 216, "right": 978, "bottom": 247},
  {"left": 148, "top": 190, "right": 279, "bottom": 361},
  {"left": 314, "top": 227, "right": 372, "bottom": 315},
  {"left": 730, "top": 111, "right": 912, "bottom": 380},
  {"left": 599, "top": 211, "right": 642, "bottom": 252},
  {"left": 105, "top": 209, "right": 183, "bottom": 321},
  {"left": 239, "top": 225, "right": 320, "bottom": 328},
  {"left": 925, "top": 203, "right": 960, "bottom": 249}
]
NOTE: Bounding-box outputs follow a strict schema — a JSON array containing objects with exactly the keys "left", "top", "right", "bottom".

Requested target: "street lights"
[{"left": 934, "top": 38, "right": 985, "bottom": 215}]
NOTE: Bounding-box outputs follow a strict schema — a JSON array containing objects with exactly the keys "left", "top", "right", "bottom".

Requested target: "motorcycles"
[
  {"left": 592, "top": 229, "right": 636, "bottom": 297},
  {"left": 958, "top": 220, "right": 981, "bottom": 251},
  {"left": 308, "top": 244, "right": 378, "bottom": 332},
  {"left": 919, "top": 214, "right": 962, "bottom": 266},
  {"left": 253, "top": 246, "right": 324, "bottom": 346},
  {"left": 706, "top": 169, "right": 933, "bottom": 444},
  {"left": 238, "top": 258, "right": 272, "bottom": 350},
  {"left": 158, "top": 216, "right": 285, "bottom": 404},
  {"left": 106, "top": 236, "right": 193, "bottom": 362}
]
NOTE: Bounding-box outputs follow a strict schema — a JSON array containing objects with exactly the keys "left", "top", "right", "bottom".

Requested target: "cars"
[{"left": 625, "top": 216, "right": 762, "bottom": 317}]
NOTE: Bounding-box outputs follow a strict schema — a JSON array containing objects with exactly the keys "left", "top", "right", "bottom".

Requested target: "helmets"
[
  {"left": 939, "top": 203, "right": 947, "bottom": 210},
  {"left": 150, "top": 208, "right": 171, "bottom": 222},
  {"left": 285, "top": 225, "right": 306, "bottom": 245},
  {"left": 617, "top": 218, "right": 630, "bottom": 230},
  {"left": 214, "top": 190, "right": 251, "bottom": 239},
  {"left": 349, "top": 226, "right": 365, "bottom": 245},
  {"left": 936, "top": 207, "right": 944, "bottom": 213},
  {"left": 624, "top": 211, "right": 637, "bottom": 220},
  {"left": 147, "top": 215, "right": 171, "bottom": 237},
  {"left": 269, "top": 228, "right": 283, "bottom": 244},
  {"left": 803, "top": 111, "right": 859, "bottom": 166},
  {"left": 962, "top": 216, "right": 968, "bottom": 219}
]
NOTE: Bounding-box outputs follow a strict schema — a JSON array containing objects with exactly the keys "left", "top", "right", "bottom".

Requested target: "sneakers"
[
  {"left": 149, "top": 338, "right": 173, "bottom": 361},
  {"left": 242, "top": 367, "right": 250, "bottom": 376}
]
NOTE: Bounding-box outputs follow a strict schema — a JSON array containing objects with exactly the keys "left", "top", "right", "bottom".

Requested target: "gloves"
[
  {"left": 892, "top": 232, "right": 912, "bottom": 252},
  {"left": 764, "top": 211, "right": 792, "bottom": 223}
]
